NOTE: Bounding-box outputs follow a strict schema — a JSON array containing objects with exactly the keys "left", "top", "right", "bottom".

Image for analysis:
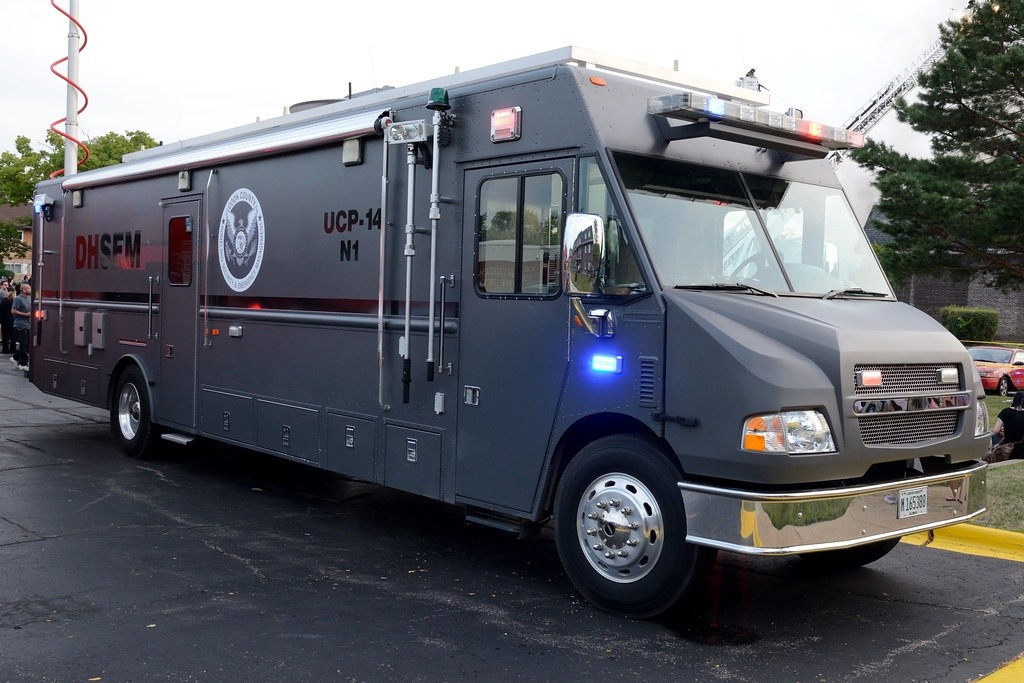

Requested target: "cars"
[{"left": 968, "top": 346, "right": 1024, "bottom": 396}]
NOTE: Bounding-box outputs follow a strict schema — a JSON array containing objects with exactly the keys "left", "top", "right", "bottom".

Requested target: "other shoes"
[
  {"left": 19, "top": 365, "right": 29, "bottom": 371},
  {"left": 10, "top": 357, "right": 17, "bottom": 366}
]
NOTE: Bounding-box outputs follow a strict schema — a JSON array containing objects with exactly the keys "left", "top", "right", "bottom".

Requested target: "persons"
[
  {"left": 0, "top": 275, "right": 31, "bottom": 371},
  {"left": 991, "top": 390, "right": 1024, "bottom": 460}
]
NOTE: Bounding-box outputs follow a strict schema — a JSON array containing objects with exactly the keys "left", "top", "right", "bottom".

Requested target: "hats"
[{"left": 7, "top": 287, "right": 14, "bottom": 292}]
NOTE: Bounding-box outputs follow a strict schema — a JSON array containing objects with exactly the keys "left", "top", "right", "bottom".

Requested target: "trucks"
[{"left": 24, "top": 44, "right": 993, "bottom": 625}]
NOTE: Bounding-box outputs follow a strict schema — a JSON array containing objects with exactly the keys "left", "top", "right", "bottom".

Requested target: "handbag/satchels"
[{"left": 982, "top": 443, "right": 1014, "bottom": 463}]
